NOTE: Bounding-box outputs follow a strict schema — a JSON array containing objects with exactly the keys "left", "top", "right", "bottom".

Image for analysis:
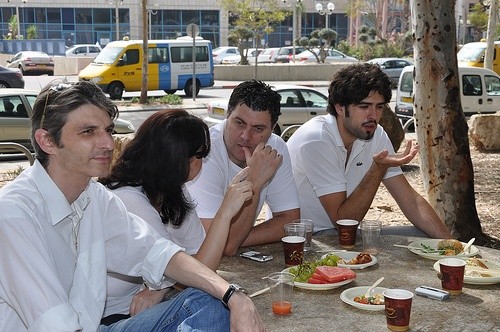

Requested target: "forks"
[
  {"left": 393, "top": 244, "right": 441, "bottom": 256},
  {"left": 249, "top": 277, "right": 290, "bottom": 298}
]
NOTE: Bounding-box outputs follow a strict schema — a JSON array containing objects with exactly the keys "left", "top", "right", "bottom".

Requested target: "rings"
[{"left": 267, "top": 146, "right": 272, "bottom": 150}]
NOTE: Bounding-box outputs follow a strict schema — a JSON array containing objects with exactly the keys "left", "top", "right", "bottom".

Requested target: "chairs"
[
  {"left": 464, "top": 84, "right": 475, "bottom": 95},
  {"left": 151, "top": 49, "right": 164, "bottom": 63},
  {"left": 15, "top": 104, "right": 29, "bottom": 118},
  {"left": 287, "top": 97, "right": 294, "bottom": 105},
  {"left": 0, "top": 102, "right": 15, "bottom": 117},
  {"left": 307, "top": 101, "right": 313, "bottom": 107}
]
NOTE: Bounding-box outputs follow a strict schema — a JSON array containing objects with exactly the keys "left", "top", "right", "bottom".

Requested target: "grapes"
[{"left": 287, "top": 254, "right": 337, "bottom": 282}]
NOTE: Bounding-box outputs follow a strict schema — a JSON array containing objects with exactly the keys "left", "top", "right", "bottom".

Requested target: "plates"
[
  {"left": 280, "top": 265, "right": 355, "bottom": 290},
  {"left": 407, "top": 240, "right": 480, "bottom": 261},
  {"left": 339, "top": 286, "right": 390, "bottom": 310},
  {"left": 320, "top": 251, "right": 378, "bottom": 270},
  {"left": 433, "top": 258, "right": 500, "bottom": 285}
]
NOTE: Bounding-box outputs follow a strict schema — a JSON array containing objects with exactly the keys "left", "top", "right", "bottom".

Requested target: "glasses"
[{"left": 39, "top": 81, "right": 86, "bottom": 130}]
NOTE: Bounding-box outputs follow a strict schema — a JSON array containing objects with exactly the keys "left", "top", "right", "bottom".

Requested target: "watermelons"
[{"left": 308, "top": 266, "right": 357, "bottom": 284}]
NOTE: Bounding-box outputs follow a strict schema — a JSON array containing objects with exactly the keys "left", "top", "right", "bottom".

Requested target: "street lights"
[{"left": 316, "top": 1, "right": 335, "bottom": 28}]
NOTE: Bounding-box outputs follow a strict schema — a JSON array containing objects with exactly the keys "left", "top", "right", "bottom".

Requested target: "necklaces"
[{"left": 345, "top": 137, "right": 357, "bottom": 150}]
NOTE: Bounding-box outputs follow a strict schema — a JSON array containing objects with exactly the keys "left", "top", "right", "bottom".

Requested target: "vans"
[
  {"left": 457, "top": 38, "right": 500, "bottom": 74},
  {"left": 63, "top": 44, "right": 103, "bottom": 58},
  {"left": 76, "top": 36, "right": 215, "bottom": 101},
  {"left": 395, "top": 64, "right": 500, "bottom": 131}
]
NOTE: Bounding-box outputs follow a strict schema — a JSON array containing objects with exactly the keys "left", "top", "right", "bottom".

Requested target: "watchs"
[{"left": 221, "top": 283, "right": 249, "bottom": 309}]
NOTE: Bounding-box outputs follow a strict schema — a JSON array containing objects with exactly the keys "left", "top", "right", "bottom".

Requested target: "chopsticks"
[{"left": 316, "top": 250, "right": 347, "bottom": 253}]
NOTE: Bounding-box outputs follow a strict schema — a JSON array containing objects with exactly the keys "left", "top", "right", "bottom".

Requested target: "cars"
[
  {"left": 0, "top": 63, "right": 26, "bottom": 88},
  {"left": 201, "top": 81, "right": 334, "bottom": 143},
  {"left": 211, "top": 46, "right": 359, "bottom": 66},
  {"left": 0, "top": 88, "right": 136, "bottom": 154},
  {"left": 5, "top": 51, "right": 56, "bottom": 77},
  {"left": 365, "top": 57, "right": 414, "bottom": 86}
]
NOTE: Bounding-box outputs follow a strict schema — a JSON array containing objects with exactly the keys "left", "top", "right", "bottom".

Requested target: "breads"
[{"left": 437, "top": 239, "right": 463, "bottom": 252}]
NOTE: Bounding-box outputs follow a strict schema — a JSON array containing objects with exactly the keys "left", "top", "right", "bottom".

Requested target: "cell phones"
[
  {"left": 240, "top": 251, "right": 273, "bottom": 262},
  {"left": 415, "top": 285, "right": 450, "bottom": 301}
]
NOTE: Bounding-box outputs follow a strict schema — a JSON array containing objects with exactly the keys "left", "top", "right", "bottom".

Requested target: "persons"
[
  {"left": 0, "top": 80, "right": 266, "bottom": 332},
  {"left": 182, "top": 79, "right": 301, "bottom": 257},
  {"left": 99, "top": 109, "right": 254, "bottom": 322},
  {"left": 266, "top": 62, "right": 454, "bottom": 240}
]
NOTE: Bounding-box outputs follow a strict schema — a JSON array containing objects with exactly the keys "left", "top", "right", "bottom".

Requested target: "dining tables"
[{"left": 164, "top": 235, "right": 500, "bottom": 332}]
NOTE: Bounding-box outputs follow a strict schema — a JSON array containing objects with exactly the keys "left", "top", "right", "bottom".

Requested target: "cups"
[
  {"left": 281, "top": 236, "right": 306, "bottom": 268},
  {"left": 293, "top": 219, "right": 314, "bottom": 251},
  {"left": 360, "top": 220, "right": 382, "bottom": 255},
  {"left": 439, "top": 258, "right": 466, "bottom": 296},
  {"left": 336, "top": 219, "right": 359, "bottom": 249},
  {"left": 382, "top": 288, "right": 414, "bottom": 332},
  {"left": 283, "top": 223, "right": 306, "bottom": 238},
  {"left": 267, "top": 272, "right": 296, "bottom": 315}
]
